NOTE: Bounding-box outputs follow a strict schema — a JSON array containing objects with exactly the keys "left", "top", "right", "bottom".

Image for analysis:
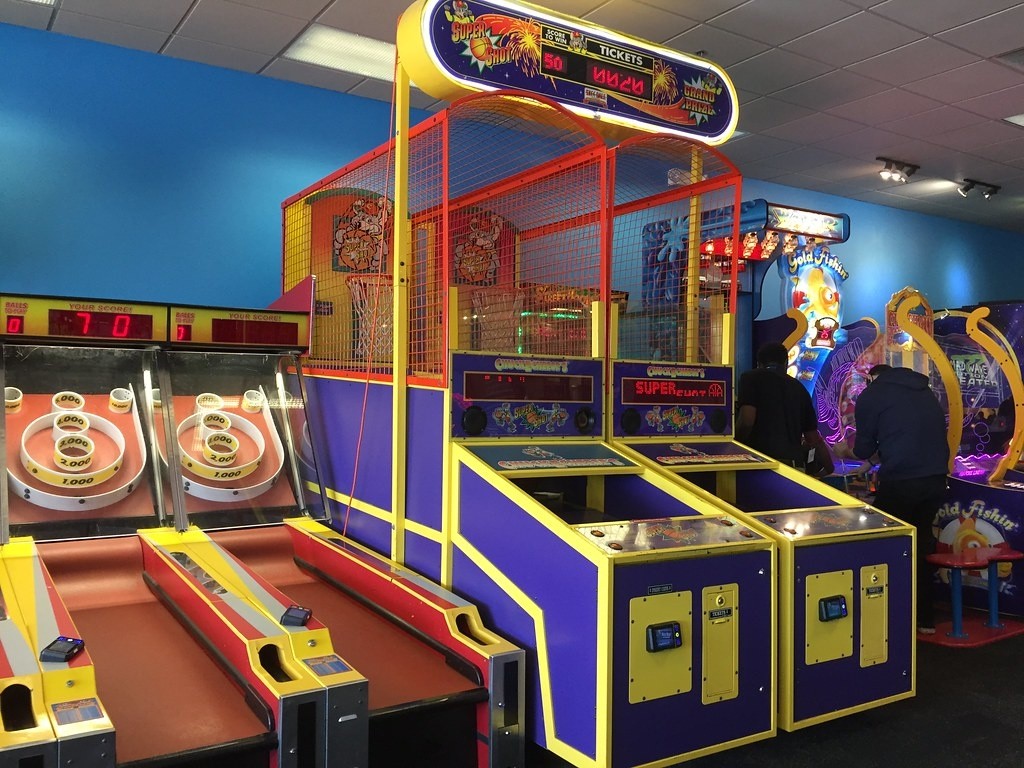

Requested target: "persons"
[
  {"left": 834, "top": 364, "right": 951, "bottom": 635},
  {"left": 733, "top": 342, "right": 818, "bottom": 474}
]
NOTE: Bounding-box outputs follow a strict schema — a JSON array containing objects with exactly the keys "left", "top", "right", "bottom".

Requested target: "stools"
[{"left": 915, "top": 548, "right": 1024, "bottom": 650}]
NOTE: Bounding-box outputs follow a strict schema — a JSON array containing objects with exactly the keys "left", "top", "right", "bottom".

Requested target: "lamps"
[
  {"left": 957, "top": 179, "right": 1001, "bottom": 200},
  {"left": 875, "top": 156, "right": 921, "bottom": 183}
]
